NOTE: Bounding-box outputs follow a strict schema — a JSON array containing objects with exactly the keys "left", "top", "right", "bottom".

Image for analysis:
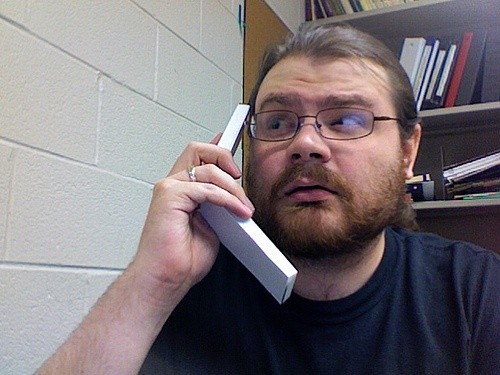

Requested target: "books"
[
  {"left": 318, "top": 1, "right": 412, "bottom": 19},
  {"left": 442, "top": 146, "right": 500, "bottom": 200}
]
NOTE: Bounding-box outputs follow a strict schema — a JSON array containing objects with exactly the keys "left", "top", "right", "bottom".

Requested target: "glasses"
[{"left": 244, "top": 106, "right": 402, "bottom": 142}]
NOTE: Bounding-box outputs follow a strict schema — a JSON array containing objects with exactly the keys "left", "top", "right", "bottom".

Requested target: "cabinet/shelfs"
[{"left": 242, "top": 1, "right": 499, "bottom": 256}]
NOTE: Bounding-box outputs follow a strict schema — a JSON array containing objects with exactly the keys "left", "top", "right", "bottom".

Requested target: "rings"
[{"left": 187, "top": 165, "right": 196, "bottom": 182}]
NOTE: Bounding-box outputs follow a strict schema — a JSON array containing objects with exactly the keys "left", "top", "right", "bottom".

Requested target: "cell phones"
[{"left": 199, "top": 104, "right": 299, "bottom": 305}]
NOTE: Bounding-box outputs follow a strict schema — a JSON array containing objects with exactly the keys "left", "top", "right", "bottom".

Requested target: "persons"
[{"left": 32, "top": 22, "right": 500, "bottom": 375}]
